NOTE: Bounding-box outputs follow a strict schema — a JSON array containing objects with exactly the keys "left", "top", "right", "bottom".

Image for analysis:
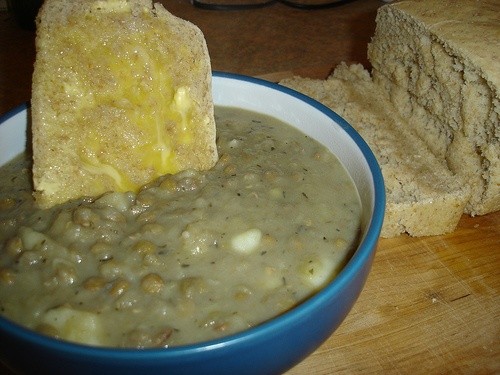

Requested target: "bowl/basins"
[{"left": 1, "top": 73, "right": 387, "bottom": 374}]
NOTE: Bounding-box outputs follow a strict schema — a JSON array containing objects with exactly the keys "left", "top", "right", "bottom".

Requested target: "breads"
[
  {"left": 31, "top": 0, "right": 220, "bottom": 209},
  {"left": 278, "top": 62, "right": 471, "bottom": 240},
  {"left": 367, "top": 0, "right": 500, "bottom": 217}
]
